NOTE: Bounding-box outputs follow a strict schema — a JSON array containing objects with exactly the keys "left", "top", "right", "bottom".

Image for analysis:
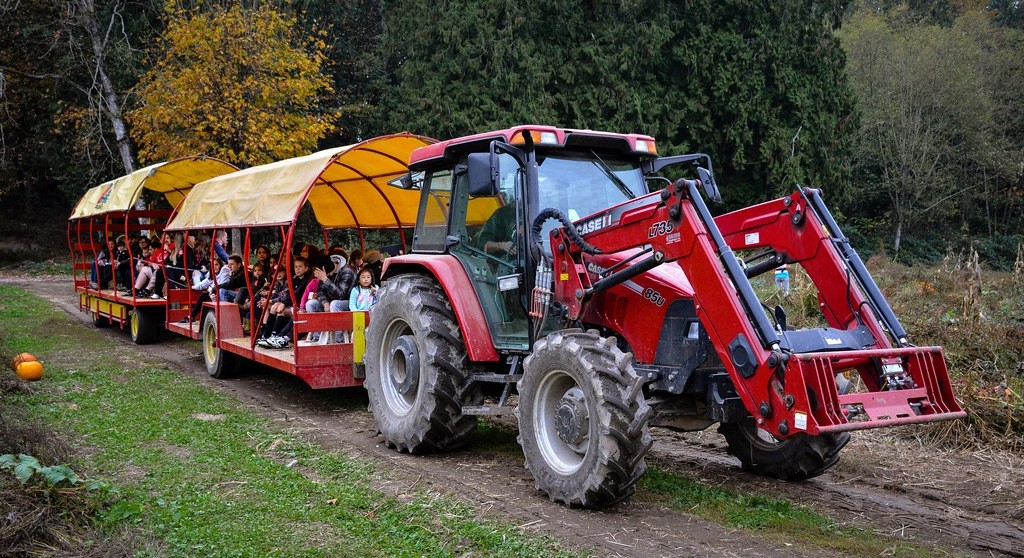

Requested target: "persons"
[
  {"left": 773, "top": 262, "right": 790, "bottom": 299},
  {"left": 94, "top": 230, "right": 384, "bottom": 351},
  {"left": 478, "top": 180, "right": 559, "bottom": 318}
]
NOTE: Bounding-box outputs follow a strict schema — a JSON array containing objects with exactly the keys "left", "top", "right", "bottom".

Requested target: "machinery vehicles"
[{"left": 68, "top": 123, "right": 970, "bottom": 508}]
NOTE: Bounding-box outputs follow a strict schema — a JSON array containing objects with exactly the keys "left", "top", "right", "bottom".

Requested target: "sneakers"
[
  {"left": 89, "top": 281, "right": 98, "bottom": 291},
  {"left": 179, "top": 316, "right": 196, "bottom": 323},
  {"left": 150, "top": 293, "right": 160, "bottom": 299},
  {"left": 126, "top": 288, "right": 138, "bottom": 296},
  {"left": 258, "top": 336, "right": 289, "bottom": 349},
  {"left": 137, "top": 289, "right": 149, "bottom": 299}
]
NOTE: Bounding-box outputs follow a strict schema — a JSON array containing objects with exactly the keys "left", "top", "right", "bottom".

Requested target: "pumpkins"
[
  {"left": 11, "top": 352, "right": 38, "bottom": 371},
  {"left": 17, "top": 361, "right": 43, "bottom": 379}
]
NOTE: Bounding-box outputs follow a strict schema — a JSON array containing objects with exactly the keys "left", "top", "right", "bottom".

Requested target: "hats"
[
  {"left": 363, "top": 250, "right": 382, "bottom": 266},
  {"left": 313, "top": 254, "right": 335, "bottom": 275}
]
